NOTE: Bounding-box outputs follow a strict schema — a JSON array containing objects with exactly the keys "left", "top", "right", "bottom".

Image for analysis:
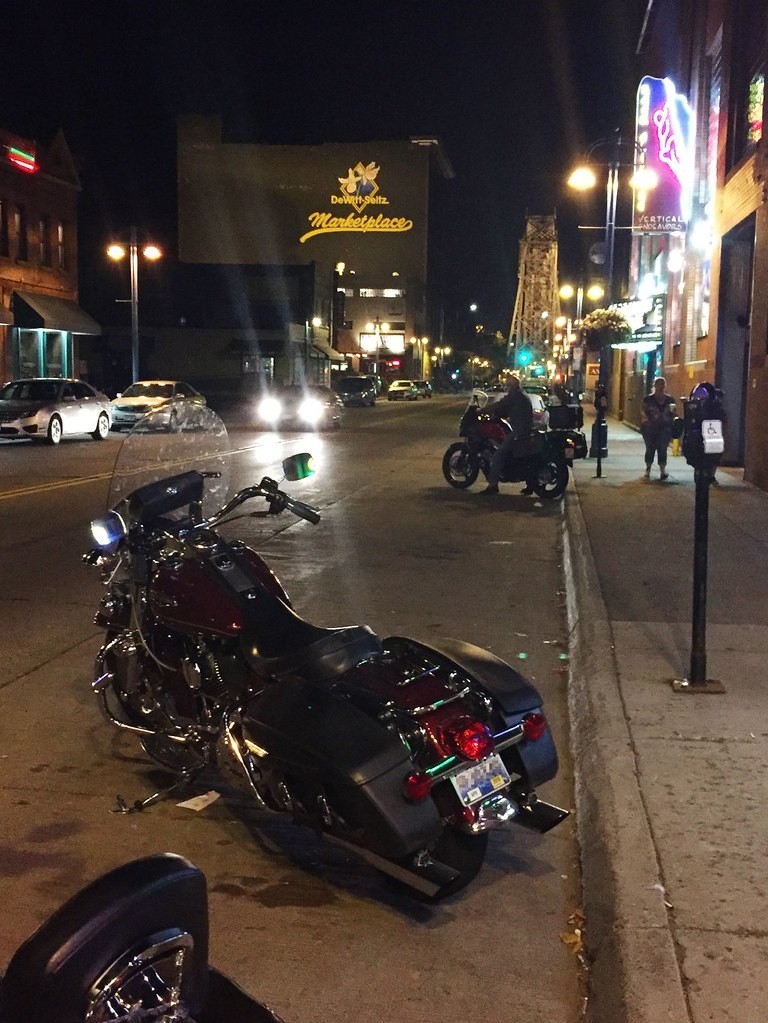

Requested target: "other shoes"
[
  {"left": 480, "top": 484, "right": 499, "bottom": 495},
  {"left": 661, "top": 473, "right": 668, "bottom": 480},
  {"left": 711, "top": 476, "right": 719, "bottom": 484},
  {"left": 520, "top": 487, "right": 533, "bottom": 495}
]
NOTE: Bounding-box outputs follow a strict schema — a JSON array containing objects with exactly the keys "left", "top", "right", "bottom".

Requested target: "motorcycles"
[
  {"left": 82, "top": 395, "right": 574, "bottom": 907},
  {"left": 440, "top": 386, "right": 588, "bottom": 498},
  {"left": 0, "top": 850, "right": 281, "bottom": 1022}
]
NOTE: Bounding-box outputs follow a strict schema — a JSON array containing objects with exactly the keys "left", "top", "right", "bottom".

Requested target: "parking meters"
[
  {"left": 593, "top": 383, "right": 608, "bottom": 477},
  {"left": 667, "top": 380, "right": 727, "bottom": 695}
]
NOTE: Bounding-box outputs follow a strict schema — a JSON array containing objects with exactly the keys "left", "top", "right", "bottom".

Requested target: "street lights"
[
  {"left": 563, "top": 122, "right": 659, "bottom": 308},
  {"left": 365, "top": 315, "right": 391, "bottom": 398},
  {"left": 104, "top": 225, "right": 162, "bottom": 385},
  {"left": 434, "top": 345, "right": 490, "bottom": 391},
  {"left": 304, "top": 316, "right": 321, "bottom": 390},
  {"left": 545, "top": 267, "right": 604, "bottom": 386},
  {"left": 409, "top": 336, "right": 429, "bottom": 380}
]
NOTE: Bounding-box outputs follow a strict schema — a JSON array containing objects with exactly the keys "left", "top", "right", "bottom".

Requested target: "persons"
[
  {"left": 642, "top": 377, "right": 677, "bottom": 481},
  {"left": 475, "top": 375, "right": 533, "bottom": 497}
]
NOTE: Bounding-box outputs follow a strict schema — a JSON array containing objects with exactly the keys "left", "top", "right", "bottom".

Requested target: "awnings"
[
  {"left": 312, "top": 344, "right": 344, "bottom": 361},
  {"left": 13, "top": 290, "right": 102, "bottom": 335}
]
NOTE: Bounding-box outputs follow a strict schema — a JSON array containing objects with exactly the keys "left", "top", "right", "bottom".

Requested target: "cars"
[
  {"left": 413, "top": 381, "right": 433, "bottom": 398},
  {"left": 388, "top": 379, "right": 418, "bottom": 400},
  {"left": 108, "top": 380, "right": 208, "bottom": 431},
  {"left": 337, "top": 375, "right": 376, "bottom": 406},
  {"left": 257, "top": 384, "right": 345, "bottom": 432},
  {"left": 491, "top": 375, "right": 550, "bottom": 432},
  {"left": 0, "top": 376, "right": 113, "bottom": 445}
]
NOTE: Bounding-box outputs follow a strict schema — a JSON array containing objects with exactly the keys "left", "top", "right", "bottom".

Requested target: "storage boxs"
[{"left": 548, "top": 404, "right": 583, "bottom": 431}]
